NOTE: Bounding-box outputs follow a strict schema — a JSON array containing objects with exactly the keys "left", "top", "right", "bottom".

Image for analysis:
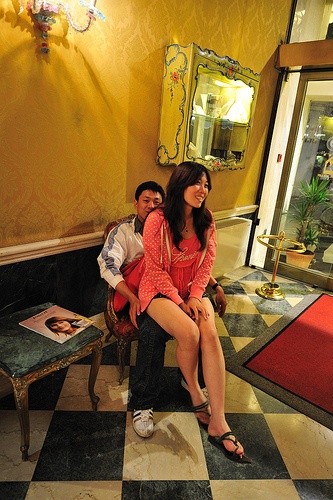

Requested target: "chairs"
[{"left": 102, "top": 214, "right": 139, "bottom": 385}]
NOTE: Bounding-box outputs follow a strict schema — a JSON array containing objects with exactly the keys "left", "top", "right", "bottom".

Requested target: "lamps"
[{"left": 26, "top": 0, "right": 106, "bottom": 53}]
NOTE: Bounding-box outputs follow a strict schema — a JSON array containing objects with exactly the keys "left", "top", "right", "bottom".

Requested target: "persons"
[
  {"left": 139, "top": 162, "right": 252, "bottom": 465},
  {"left": 45, "top": 315, "right": 83, "bottom": 337},
  {"left": 96, "top": 180, "right": 227, "bottom": 434}
]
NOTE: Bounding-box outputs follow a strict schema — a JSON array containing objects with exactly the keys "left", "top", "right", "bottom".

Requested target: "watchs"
[{"left": 212, "top": 281, "right": 222, "bottom": 290}]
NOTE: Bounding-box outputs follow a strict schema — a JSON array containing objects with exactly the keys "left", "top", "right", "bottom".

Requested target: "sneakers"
[
  {"left": 179, "top": 379, "right": 208, "bottom": 398},
  {"left": 132, "top": 408, "right": 154, "bottom": 439}
]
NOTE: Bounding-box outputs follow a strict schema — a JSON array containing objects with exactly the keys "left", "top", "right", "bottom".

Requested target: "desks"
[{"left": 0, "top": 302, "right": 104, "bottom": 461}]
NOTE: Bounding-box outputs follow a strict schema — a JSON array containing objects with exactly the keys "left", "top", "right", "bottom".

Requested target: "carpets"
[{"left": 227, "top": 288, "right": 333, "bottom": 431}]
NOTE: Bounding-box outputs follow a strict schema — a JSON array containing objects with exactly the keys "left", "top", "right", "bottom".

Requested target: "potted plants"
[{"left": 282, "top": 176, "right": 333, "bottom": 268}]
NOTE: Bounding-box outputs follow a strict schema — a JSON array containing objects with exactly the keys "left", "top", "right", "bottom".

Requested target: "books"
[{"left": 18, "top": 304, "right": 95, "bottom": 344}]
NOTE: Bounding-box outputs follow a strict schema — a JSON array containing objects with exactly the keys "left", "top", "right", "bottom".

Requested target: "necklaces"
[{"left": 184, "top": 225, "right": 189, "bottom": 232}]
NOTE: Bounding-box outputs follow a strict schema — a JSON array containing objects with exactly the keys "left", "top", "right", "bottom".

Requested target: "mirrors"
[{"left": 157, "top": 41, "right": 261, "bottom": 171}]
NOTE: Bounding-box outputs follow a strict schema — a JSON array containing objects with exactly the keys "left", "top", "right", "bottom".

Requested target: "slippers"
[
  {"left": 194, "top": 398, "right": 212, "bottom": 428},
  {"left": 209, "top": 430, "right": 251, "bottom": 464}
]
